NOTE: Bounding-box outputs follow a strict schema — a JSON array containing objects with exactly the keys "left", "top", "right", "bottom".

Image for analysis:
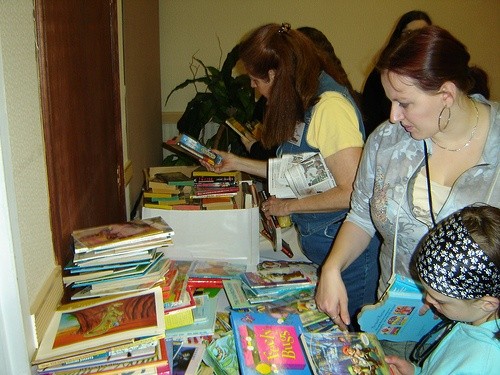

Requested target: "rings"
[
  {"left": 263, "top": 206, "right": 266, "bottom": 212},
  {"left": 331, "top": 315, "right": 340, "bottom": 320}
]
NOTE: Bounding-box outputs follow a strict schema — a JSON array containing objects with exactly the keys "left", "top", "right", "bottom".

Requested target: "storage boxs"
[{"left": 140, "top": 170, "right": 260, "bottom": 261}]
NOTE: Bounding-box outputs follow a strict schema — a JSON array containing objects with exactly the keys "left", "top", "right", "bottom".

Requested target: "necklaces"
[{"left": 428, "top": 96, "right": 480, "bottom": 153}]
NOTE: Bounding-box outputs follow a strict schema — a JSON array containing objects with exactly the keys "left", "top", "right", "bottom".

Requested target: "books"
[
  {"left": 164, "top": 133, "right": 224, "bottom": 168},
  {"left": 225, "top": 116, "right": 264, "bottom": 146},
  {"left": 354, "top": 292, "right": 449, "bottom": 343},
  {"left": 29, "top": 166, "right": 396, "bottom": 375},
  {"left": 377, "top": 273, "right": 424, "bottom": 305},
  {"left": 296, "top": 331, "right": 396, "bottom": 375}
]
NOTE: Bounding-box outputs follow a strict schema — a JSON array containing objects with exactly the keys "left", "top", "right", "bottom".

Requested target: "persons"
[
  {"left": 360, "top": 10, "right": 434, "bottom": 141},
  {"left": 314, "top": 23, "right": 500, "bottom": 368},
  {"left": 381, "top": 204, "right": 499, "bottom": 375},
  {"left": 239, "top": 26, "right": 371, "bottom": 184},
  {"left": 190, "top": 22, "right": 383, "bottom": 335}
]
